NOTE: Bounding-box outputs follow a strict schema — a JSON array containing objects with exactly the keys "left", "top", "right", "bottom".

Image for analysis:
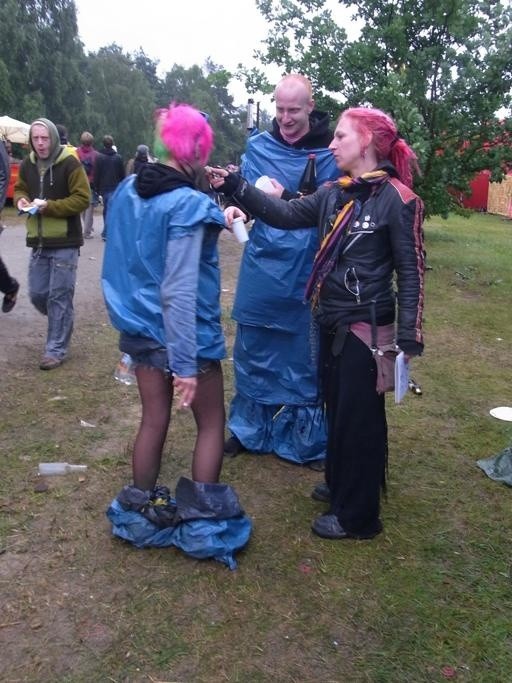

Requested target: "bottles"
[
  {"left": 408, "top": 378, "right": 422, "bottom": 397},
  {"left": 39, "top": 459, "right": 86, "bottom": 477},
  {"left": 115, "top": 353, "right": 136, "bottom": 385}
]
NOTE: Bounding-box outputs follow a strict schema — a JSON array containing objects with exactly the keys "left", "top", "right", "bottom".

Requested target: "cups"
[
  {"left": 231, "top": 216, "right": 249, "bottom": 244},
  {"left": 254, "top": 174, "right": 276, "bottom": 194}
]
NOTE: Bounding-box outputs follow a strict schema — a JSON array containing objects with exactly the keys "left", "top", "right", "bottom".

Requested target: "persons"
[
  {"left": 102, "top": 103, "right": 233, "bottom": 504},
  {"left": 57, "top": 125, "right": 153, "bottom": 241},
  {"left": 0, "top": 138, "right": 19, "bottom": 312},
  {"left": 11, "top": 116, "right": 95, "bottom": 370},
  {"left": 223, "top": 71, "right": 342, "bottom": 474},
  {"left": 206, "top": 103, "right": 428, "bottom": 542}
]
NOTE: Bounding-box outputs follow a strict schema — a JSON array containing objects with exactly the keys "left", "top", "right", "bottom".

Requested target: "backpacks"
[{"left": 80, "top": 157, "right": 93, "bottom": 176}]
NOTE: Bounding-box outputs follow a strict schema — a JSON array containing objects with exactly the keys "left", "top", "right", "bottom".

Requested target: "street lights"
[{"left": 245, "top": 96, "right": 262, "bottom": 135}]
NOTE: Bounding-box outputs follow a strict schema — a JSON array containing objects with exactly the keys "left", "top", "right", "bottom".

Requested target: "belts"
[{"left": 328, "top": 322, "right": 350, "bottom": 358}]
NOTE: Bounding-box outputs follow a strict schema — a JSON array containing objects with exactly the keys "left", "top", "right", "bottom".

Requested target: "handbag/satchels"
[{"left": 348, "top": 321, "right": 402, "bottom": 397}]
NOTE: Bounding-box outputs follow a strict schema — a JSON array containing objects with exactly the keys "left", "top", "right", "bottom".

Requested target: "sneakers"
[
  {"left": 2, "top": 276, "right": 20, "bottom": 313},
  {"left": 101, "top": 232, "right": 107, "bottom": 241},
  {"left": 309, "top": 512, "right": 350, "bottom": 540},
  {"left": 39, "top": 355, "right": 62, "bottom": 371},
  {"left": 84, "top": 229, "right": 95, "bottom": 240},
  {"left": 308, "top": 458, "right": 327, "bottom": 472},
  {"left": 310, "top": 481, "right": 337, "bottom": 503},
  {"left": 223, "top": 434, "right": 245, "bottom": 458}
]
